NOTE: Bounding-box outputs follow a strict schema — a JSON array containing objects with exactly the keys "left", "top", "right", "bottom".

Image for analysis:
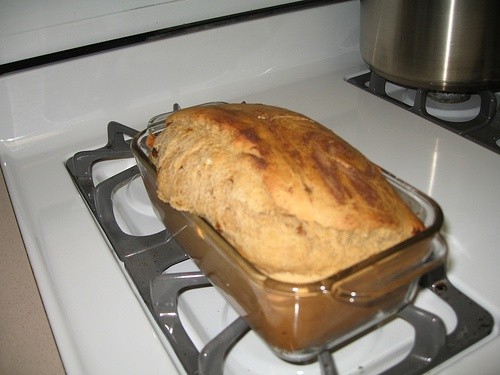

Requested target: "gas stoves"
[{"left": 0, "top": 2, "right": 499, "bottom": 375}]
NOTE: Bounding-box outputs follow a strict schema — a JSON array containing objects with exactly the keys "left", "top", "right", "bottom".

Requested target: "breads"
[{"left": 155, "top": 101, "right": 426, "bottom": 283}]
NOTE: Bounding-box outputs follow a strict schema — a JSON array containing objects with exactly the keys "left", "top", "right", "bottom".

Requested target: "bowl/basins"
[{"left": 130, "top": 100, "right": 451, "bottom": 364}]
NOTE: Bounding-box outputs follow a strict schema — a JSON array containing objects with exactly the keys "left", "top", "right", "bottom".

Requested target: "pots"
[{"left": 353, "top": 1, "right": 499, "bottom": 92}]
